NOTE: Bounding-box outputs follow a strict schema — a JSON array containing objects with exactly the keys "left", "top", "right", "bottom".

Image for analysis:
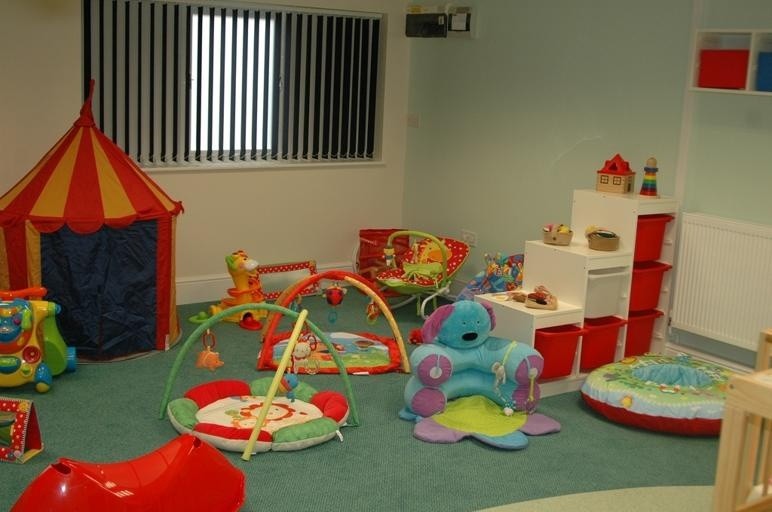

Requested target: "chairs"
[{"left": 365, "top": 229, "right": 469, "bottom": 325}]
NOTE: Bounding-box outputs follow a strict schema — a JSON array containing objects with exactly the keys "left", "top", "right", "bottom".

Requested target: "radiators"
[{"left": 671, "top": 212, "right": 772, "bottom": 353}]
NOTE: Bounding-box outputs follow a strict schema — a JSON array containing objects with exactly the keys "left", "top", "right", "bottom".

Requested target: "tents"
[{"left": 1, "top": 77, "right": 186, "bottom": 360}]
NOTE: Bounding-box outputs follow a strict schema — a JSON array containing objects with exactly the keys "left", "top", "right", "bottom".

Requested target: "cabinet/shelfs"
[{"left": 473, "top": 189, "right": 679, "bottom": 401}]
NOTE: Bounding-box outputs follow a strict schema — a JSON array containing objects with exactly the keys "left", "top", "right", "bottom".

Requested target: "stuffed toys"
[
  {"left": 293, "top": 342, "right": 311, "bottom": 375},
  {"left": 279, "top": 374, "right": 298, "bottom": 403},
  {"left": 401, "top": 297, "right": 559, "bottom": 452},
  {"left": 322, "top": 286, "right": 347, "bottom": 308},
  {"left": 195, "top": 350, "right": 224, "bottom": 372},
  {"left": 365, "top": 303, "right": 379, "bottom": 321}
]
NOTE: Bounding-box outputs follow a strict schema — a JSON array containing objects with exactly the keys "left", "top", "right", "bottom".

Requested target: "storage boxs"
[
  {"left": 536, "top": 214, "right": 672, "bottom": 382},
  {"left": 701, "top": 49, "right": 749, "bottom": 87}
]
